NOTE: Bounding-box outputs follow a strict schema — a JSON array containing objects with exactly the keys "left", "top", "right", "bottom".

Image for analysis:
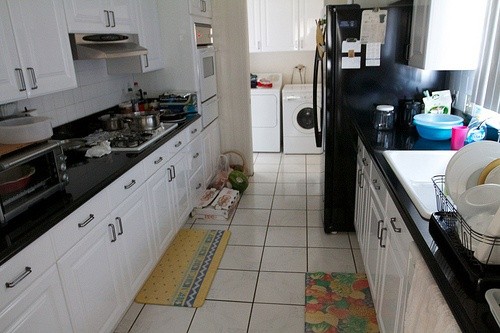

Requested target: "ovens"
[
  {"left": 191, "top": 23, "right": 217, "bottom": 104},
  {"left": 0, "top": 138, "right": 70, "bottom": 225}
]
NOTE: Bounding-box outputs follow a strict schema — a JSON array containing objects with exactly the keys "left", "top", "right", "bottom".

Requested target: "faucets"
[{"left": 476, "top": 113, "right": 500, "bottom": 143}]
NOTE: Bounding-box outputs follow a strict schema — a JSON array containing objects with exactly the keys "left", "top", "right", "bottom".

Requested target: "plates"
[{"left": 445, "top": 138, "right": 500, "bottom": 204}]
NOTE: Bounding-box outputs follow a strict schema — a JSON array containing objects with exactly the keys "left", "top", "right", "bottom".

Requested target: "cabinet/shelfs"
[
  {"left": 0, "top": 233, "right": 73, "bottom": 333},
  {"left": 106, "top": 0, "right": 163, "bottom": 74},
  {"left": 48, "top": 159, "right": 155, "bottom": 333},
  {"left": 184, "top": 120, "right": 208, "bottom": 215},
  {"left": 0, "top": 0, "right": 77, "bottom": 106},
  {"left": 408, "top": 0, "right": 487, "bottom": 73},
  {"left": 354, "top": 136, "right": 463, "bottom": 333},
  {"left": 62, "top": 0, "right": 139, "bottom": 34},
  {"left": 145, "top": 121, "right": 189, "bottom": 255}
]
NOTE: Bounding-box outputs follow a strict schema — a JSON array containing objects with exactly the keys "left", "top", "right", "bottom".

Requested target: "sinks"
[{"left": 382, "top": 149, "right": 459, "bottom": 220}]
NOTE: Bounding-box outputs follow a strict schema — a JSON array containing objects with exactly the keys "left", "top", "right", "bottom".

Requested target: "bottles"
[
  {"left": 372, "top": 104, "right": 397, "bottom": 130},
  {"left": 450, "top": 126, "right": 469, "bottom": 150},
  {"left": 120, "top": 81, "right": 150, "bottom": 112}
]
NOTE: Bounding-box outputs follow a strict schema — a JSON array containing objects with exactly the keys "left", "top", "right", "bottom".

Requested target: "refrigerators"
[{"left": 312, "top": 3, "right": 447, "bottom": 234}]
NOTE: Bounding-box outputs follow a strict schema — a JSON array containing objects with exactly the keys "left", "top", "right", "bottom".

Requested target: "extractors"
[{"left": 67, "top": 32, "right": 148, "bottom": 61}]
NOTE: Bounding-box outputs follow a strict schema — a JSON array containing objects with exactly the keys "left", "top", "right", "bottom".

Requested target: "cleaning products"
[{"left": 463, "top": 106, "right": 488, "bottom": 147}]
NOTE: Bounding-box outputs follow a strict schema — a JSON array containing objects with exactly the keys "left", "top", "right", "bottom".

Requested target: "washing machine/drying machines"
[{"left": 282, "top": 82, "right": 324, "bottom": 155}]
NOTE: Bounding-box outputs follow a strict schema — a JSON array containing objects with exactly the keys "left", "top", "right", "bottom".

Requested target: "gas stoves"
[{"left": 73, "top": 119, "right": 178, "bottom": 152}]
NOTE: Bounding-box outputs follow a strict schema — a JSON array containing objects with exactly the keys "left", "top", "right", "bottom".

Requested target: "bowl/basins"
[
  {"left": 456, "top": 184, "right": 500, "bottom": 250},
  {"left": 413, "top": 112, "right": 464, "bottom": 141}
]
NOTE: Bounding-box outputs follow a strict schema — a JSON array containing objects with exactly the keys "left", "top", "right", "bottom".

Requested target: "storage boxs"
[{"left": 190, "top": 188, "right": 241, "bottom": 222}]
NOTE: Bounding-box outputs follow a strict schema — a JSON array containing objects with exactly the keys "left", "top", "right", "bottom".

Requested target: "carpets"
[
  {"left": 134, "top": 228, "right": 232, "bottom": 307},
  {"left": 305, "top": 273, "right": 380, "bottom": 333}
]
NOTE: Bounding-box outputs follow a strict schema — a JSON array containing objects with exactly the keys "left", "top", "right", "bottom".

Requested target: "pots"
[
  {"left": 98, "top": 110, "right": 124, "bottom": 131},
  {"left": 124, "top": 111, "right": 166, "bottom": 132}
]
